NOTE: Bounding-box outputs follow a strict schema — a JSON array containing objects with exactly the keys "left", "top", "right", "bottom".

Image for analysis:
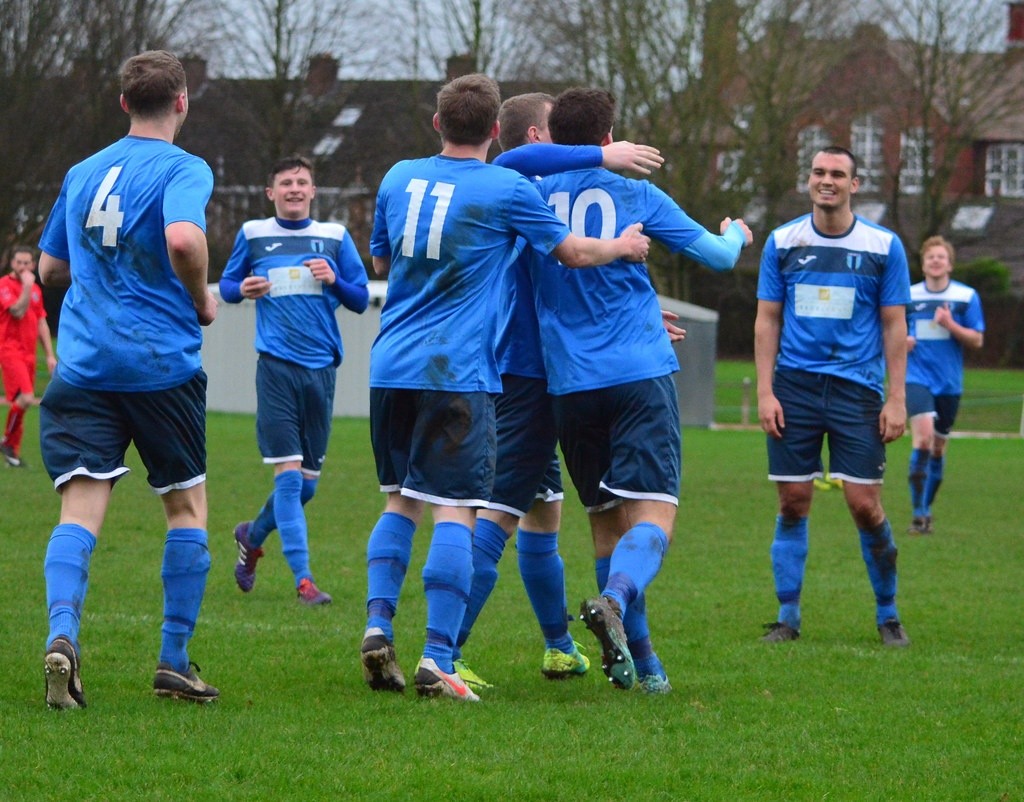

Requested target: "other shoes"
[{"left": 1, "top": 445, "right": 24, "bottom": 468}]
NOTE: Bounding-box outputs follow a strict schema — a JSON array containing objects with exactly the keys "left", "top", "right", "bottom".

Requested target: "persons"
[
  {"left": 905, "top": 235, "right": 985, "bottom": 535},
  {"left": 754, "top": 146, "right": 910, "bottom": 649},
  {"left": 0, "top": 245, "right": 59, "bottom": 468},
  {"left": 217, "top": 153, "right": 369, "bottom": 604},
  {"left": 359, "top": 70, "right": 754, "bottom": 695},
  {"left": 39, "top": 48, "right": 221, "bottom": 710}
]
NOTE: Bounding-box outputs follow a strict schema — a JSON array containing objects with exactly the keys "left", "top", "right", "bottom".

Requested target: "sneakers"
[
  {"left": 359, "top": 627, "right": 405, "bottom": 696},
  {"left": 415, "top": 654, "right": 480, "bottom": 702},
  {"left": 154, "top": 661, "right": 220, "bottom": 700},
  {"left": 640, "top": 672, "right": 672, "bottom": 697},
  {"left": 414, "top": 652, "right": 491, "bottom": 690},
  {"left": 44, "top": 638, "right": 86, "bottom": 711},
  {"left": 908, "top": 516, "right": 933, "bottom": 536},
  {"left": 580, "top": 595, "right": 636, "bottom": 691},
  {"left": 234, "top": 522, "right": 264, "bottom": 590},
  {"left": 542, "top": 640, "right": 590, "bottom": 679},
  {"left": 759, "top": 621, "right": 799, "bottom": 644},
  {"left": 296, "top": 580, "right": 332, "bottom": 605},
  {"left": 877, "top": 621, "right": 909, "bottom": 648}
]
